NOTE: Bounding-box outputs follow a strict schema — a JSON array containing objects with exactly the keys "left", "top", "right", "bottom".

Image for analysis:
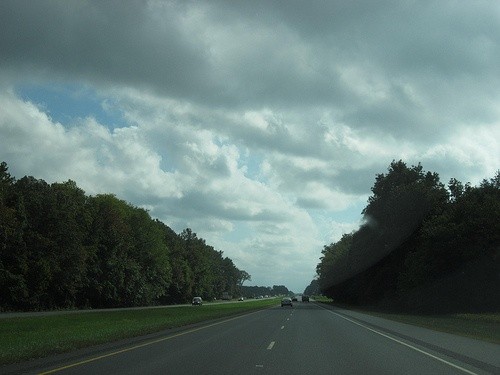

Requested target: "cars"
[
  {"left": 280, "top": 297, "right": 292, "bottom": 307},
  {"left": 192, "top": 297, "right": 202, "bottom": 306}
]
